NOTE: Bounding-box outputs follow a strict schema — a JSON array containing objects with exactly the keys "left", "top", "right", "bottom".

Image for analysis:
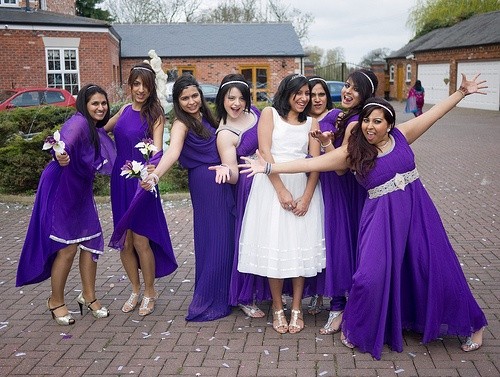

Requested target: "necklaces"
[{"left": 377, "top": 138, "right": 390, "bottom": 149}]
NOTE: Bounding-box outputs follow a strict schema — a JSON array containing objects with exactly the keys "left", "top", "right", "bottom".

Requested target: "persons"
[
  {"left": 144, "top": 49, "right": 169, "bottom": 104},
  {"left": 238, "top": 71, "right": 489, "bottom": 359},
  {"left": 237, "top": 73, "right": 328, "bottom": 335},
  {"left": 103, "top": 63, "right": 178, "bottom": 317},
  {"left": 406, "top": 79, "right": 425, "bottom": 117},
  {"left": 208, "top": 75, "right": 287, "bottom": 318},
  {"left": 16, "top": 83, "right": 122, "bottom": 325},
  {"left": 136, "top": 77, "right": 236, "bottom": 321},
  {"left": 302, "top": 75, "right": 345, "bottom": 315},
  {"left": 310, "top": 68, "right": 384, "bottom": 335}
]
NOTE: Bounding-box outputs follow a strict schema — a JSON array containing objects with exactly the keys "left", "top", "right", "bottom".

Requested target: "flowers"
[
  {"left": 135, "top": 137, "right": 158, "bottom": 165},
  {"left": 120, "top": 160, "right": 157, "bottom": 198},
  {"left": 42, "top": 130, "right": 65, "bottom": 154}
]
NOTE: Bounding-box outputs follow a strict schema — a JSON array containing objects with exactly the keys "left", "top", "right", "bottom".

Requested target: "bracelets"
[
  {"left": 150, "top": 173, "right": 159, "bottom": 184},
  {"left": 265, "top": 162, "right": 271, "bottom": 176},
  {"left": 321, "top": 140, "right": 332, "bottom": 149},
  {"left": 219, "top": 164, "right": 232, "bottom": 176},
  {"left": 457, "top": 85, "right": 468, "bottom": 96}
]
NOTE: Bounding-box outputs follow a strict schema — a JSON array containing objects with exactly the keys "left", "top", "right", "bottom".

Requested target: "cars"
[
  {"left": 198, "top": 84, "right": 219, "bottom": 104},
  {"left": 0, "top": 88, "right": 77, "bottom": 113},
  {"left": 247, "top": 80, "right": 268, "bottom": 101},
  {"left": 326, "top": 80, "right": 346, "bottom": 103}
]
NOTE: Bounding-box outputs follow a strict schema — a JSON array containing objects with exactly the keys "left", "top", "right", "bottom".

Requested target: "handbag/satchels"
[{"left": 405, "top": 95, "right": 418, "bottom": 113}]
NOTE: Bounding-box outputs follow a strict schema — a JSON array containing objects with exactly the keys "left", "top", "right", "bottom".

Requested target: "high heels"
[
  {"left": 239, "top": 304, "right": 264, "bottom": 317},
  {"left": 270, "top": 305, "right": 288, "bottom": 333},
  {"left": 138, "top": 290, "right": 159, "bottom": 316},
  {"left": 341, "top": 330, "right": 357, "bottom": 348},
  {"left": 122, "top": 291, "right": 142, "bottom": 312},
  {"left": 308, "top": 294, "right": 323, "bottom": 315},
  {"left": 281, "top": 294, "right": 288, "bottom": 310},
  {"left": 461, "top": 326, "right": 485, "bottom": 351},
  {"left": 77, "top": 292, "right": 110, "bottom": 318},
  {"left": 47, "top": 296, "right": 75, "bottom": 325},
  {"left": 289, "top": 307, "right": 304, "bottom": 334},
  {"left": 319, "top": 310, "right": 344, "bottom": 334}
]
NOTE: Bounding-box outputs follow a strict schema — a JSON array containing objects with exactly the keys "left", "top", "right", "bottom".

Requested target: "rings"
[{"left": 289, "top": 204, "right": 291, "bottom": 207}]
